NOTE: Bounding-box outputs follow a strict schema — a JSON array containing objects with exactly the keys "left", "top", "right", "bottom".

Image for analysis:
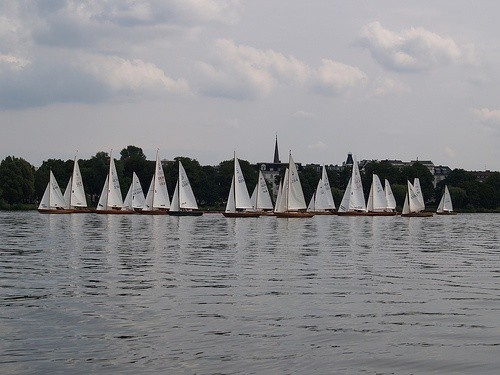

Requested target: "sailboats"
[
  {"left": 249, "top": 169, "right": 276, "bottom": 216},
  {"left": 139, "top": 148, "right": 171, "bottom": 215},
  {"left": 221, "top": 150, "right": 263, "bottom": 218},
  {"left": 272, "top": 150, "right": 315, "bottom": 218},
  {"left": 119, "top": 172, "right": 148, "bottom": 215},
  {"left": 400, "top": 176, "right": 433, "bottom": 218},
  {"left": 434, "top": 184, "right": 458, "bottom": 215},
  {"left": 166, "top": 160, "right": 204, "bottom": 216},
  {"left": 37, "top": 169, "right": 73, "bottom": 214},
  {"left": 305, "top": 163, "right": 337, "bottom": 215},
  {"left": 336, "top": 154, "right": 367, "bottom": 216},
  {"left": 366, "top": 170, "right": 401, "bottom": 216},
  {"left": 63, "top": 155, "right": 91, "bottom": 213},
  {"left": 93, "top": 149, "right": 123, "bottom": 214}
]
